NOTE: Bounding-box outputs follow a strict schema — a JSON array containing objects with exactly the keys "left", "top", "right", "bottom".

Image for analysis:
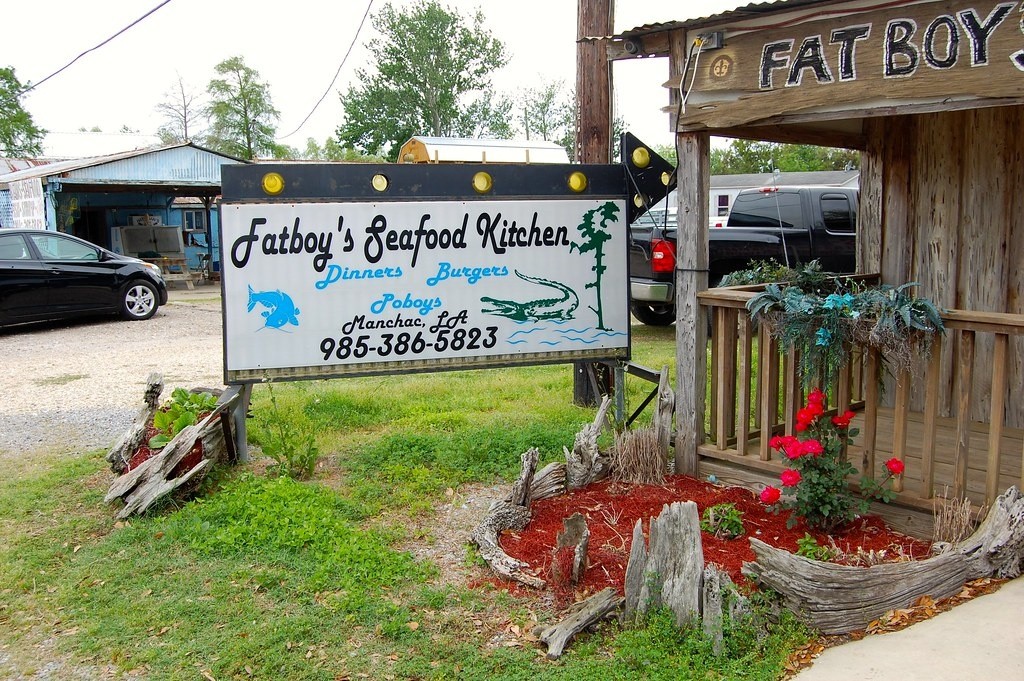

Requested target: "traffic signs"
[{"left": 214, "top": 128, "right": 677, "bottom": 386}]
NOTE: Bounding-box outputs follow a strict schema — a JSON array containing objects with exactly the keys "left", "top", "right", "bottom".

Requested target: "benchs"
[{"left": 177, "top": 270, "right": 202, "bottom": 280}]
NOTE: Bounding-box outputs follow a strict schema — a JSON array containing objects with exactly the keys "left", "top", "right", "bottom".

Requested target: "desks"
[{"left": 140, "top": 257, "right": 196, "bottom": 290}]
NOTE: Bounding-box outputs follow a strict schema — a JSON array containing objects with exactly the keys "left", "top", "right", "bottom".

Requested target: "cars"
[{"left": 0, "top": 227, "right": 168, "bottom": 329}]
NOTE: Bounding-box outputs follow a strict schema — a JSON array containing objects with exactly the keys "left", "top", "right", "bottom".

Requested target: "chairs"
[{"left": 190, "top": 253, "right": 215, "bottom": 285}]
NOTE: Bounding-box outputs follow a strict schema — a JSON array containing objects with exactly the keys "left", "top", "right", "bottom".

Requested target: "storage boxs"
[{"left": 127, "top": 215, "right": 162, "bottom": 226}]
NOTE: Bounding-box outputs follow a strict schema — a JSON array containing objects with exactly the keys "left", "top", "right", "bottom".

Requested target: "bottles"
[{"left": 144, "top": 213, "right": 149, "bottom": 225}]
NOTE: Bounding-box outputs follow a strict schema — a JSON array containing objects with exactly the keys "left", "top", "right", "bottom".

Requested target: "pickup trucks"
[
  {"left": 633, "top": 208, "right": 730, "bottom": 227},
  {"left": 629, "top": 185, "right": 859, "bottom": 339}
]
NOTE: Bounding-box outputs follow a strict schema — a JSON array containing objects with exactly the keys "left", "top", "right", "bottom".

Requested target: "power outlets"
[{"left": 697, "top": 31, "right": 723, "bottom": 50}]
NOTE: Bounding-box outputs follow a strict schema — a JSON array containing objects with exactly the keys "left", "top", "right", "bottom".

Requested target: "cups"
[{"left": 148, "top": 220, "right": 153, "bottom": 226}]
